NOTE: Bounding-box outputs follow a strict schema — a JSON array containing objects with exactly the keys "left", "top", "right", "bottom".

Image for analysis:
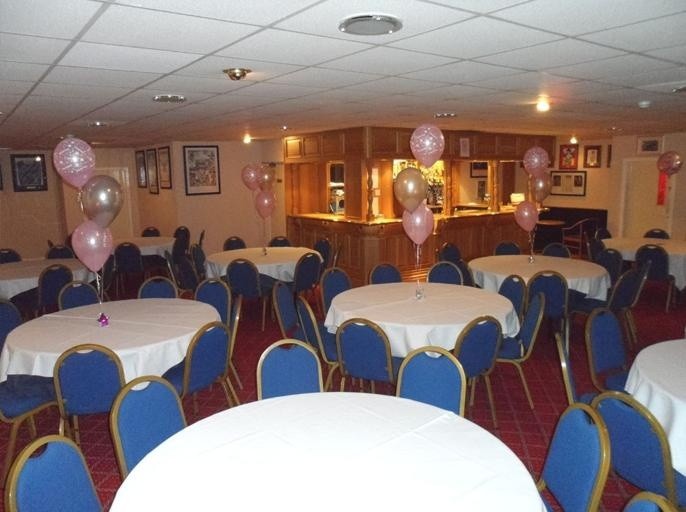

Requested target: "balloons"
[
  {"left": 78, "top": 175, "right": 123, "bottom": 227},
  {"left": 394, "top": 168, "right": 429, "bottom": 214},
  {"left": 532, "top": 173, "right": 553, "bottom": 203},
  {"left": 71, "top": 221, "right": 113, "bottom": 273},
  {"left": 254, "top": 191, "right": 277, "bottom": 221},
  {"left": 523, "top": 146, "right": 549, "bottom": 176},
  {"left": 401, "top": 204, "right": 435, "bottom": 245},
  {"left": 255, "top": 167, "right": 276, "bottom": 193},
  {"left": 657, "top": 149, "right": 684, "bottom": 176},
  {"left": 515, "top": 200, "right": 538, "bottom": 232},
  {"left": 52, "top": 137, "right": 94, "bottom": 189},
  {"left": 410, "top": 125, "right": 446, "bottom": 168},
  {"left": 240, "top": 164, "right": 260, "bottom": 191}
]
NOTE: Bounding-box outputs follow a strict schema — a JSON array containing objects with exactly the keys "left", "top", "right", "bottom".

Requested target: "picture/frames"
[
  {"left": 10, "top": 154, "right": 48, "bottom": 192},
  {"left": 470, "top": 162, "right": 487, "bottom": 178},
  {"left": 135, "top": 146, "right": 172, "bottom": 194},
  {"left": 550, "top": 171, "right": 587, "bottom": 196},
  {"left": 183, "top": 145, "right": 221, "bottom": 196}
]
